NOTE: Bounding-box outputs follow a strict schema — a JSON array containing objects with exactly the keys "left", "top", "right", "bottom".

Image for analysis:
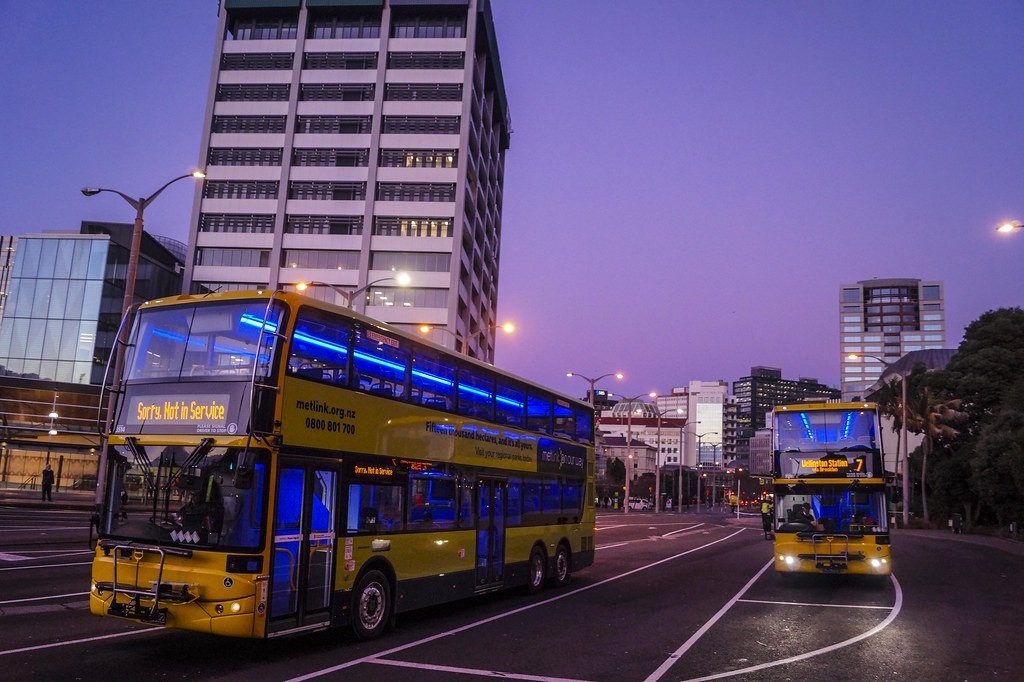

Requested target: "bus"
[
  {"left": 89, "top": 290, "right": 596, "bottom": 643},
  {"left": 770, "top": 402, "right": 891, "bottom": 575}
]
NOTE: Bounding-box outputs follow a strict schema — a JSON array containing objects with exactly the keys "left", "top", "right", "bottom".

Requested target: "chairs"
[
  {"left": 201, "top": 477, "right": 225, "bottom": 539},
  {"left": 360, "top": 384, "right": 366, "bottom": 390},
  {"left": 781, "top": 438, "right": 796, "bottom": 443},
  {"left": 838, "top": 436, "right": 856, "bottom": 443},
  {"left": 528, "top": 422, "right": 540, "bottom": 431},
  {"left": 788, "top": 504, "right": 802, "bottom": 522},
  {"left": 449, "top": 402, "right": 469, "bottom": 415},
  {"left": 400, "top": 390, "right": 421, "bottom": 404},
  {"left": 471, "top": 407, "right": 490, "bottom": 420},
  {"left": 288, "top": 363, "right": 293, "bottom": 372},
  {"left": 369, "top": 382, "right": 392, "bottom": 397},
  {"left": 322, "top": 372, "right": 332, "bottom": 382},
  {"left": 425, "top": 397, "right": 446, "bottom": 410},
  {"left": 798, "top": 438, "right": 812, "bottom": 443},
  {"left": 508, "top": 418, "right": 519, "bottom": 425},
  {"left": 336, "top": 374, "right": 360, "bottom": 389},
  {"left": 297, "top": 362, "right": 324, "bottom": 380},
  {"left": 852, "top": 511, "right": 868, "bottom": 523},
  {"left": 497, "top": 415, "right": 507, "bottom": 424},
  {"left": 856, "top": 436, "right": 874, "bottom": 444}
]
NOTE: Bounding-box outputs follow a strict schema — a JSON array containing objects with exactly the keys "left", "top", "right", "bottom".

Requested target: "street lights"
[
  {"left": 636, "top": 409, "right": 683, "bottom": 513},
  {"left": 608, "top": 393, "right": 656, "bottom": 513},
  {"left": 421, "top": 323, "right": 515, "bottom": 354},
  {"left": 703, "top": 442, "right": 726, "bottom": 507},
  {"left": 849, "top": 354, "right": 909, "bottom": 526},
  {"left": 566, "top": 373, "right": 623, "bottom": 403},
  {"left": 662, "top": 421, "right": 701, "bottom": 512},
  {"left": 81, "top": 169, "right": 207, "bottom": 504},
  {"left": 682, "top": 431, "right": 718, "bottom": 513},
  {"left": 297, "top": 276, "right": 410, "bottom": 309}
]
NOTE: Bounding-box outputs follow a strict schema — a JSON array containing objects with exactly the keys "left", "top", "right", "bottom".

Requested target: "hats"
[{"left": 802, "top": 503, "right": 811, "bottom": 510}]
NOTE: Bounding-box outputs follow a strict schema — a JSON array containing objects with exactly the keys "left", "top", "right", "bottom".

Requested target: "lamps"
[
  {"left": 1, "top": 442, "right": 8, "bottom": 449},
  {"left": 48, "top": 412, "right": 59, "bottom": 418},
  {"left": 48, "top": 430, "right": 57, "bottom": 435},
  {"left": 89, "top": 448, "right": 96, "bottom": 455}
]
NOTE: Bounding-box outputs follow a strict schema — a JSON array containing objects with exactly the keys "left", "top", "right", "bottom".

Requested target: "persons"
[
  {"left": 42, "top": 464, "right": 55, "bottom": 502},
  {"left": 603, "top": 494, "right": 609, "bottom": 507},
  {"left": 796, "top": 503, "right": 818, "bottom": 525},
  {"left": 171, "top": 488, "right": 207, "bottom": 529}
]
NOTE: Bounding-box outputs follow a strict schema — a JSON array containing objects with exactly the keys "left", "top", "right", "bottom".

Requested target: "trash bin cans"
[{"left": 951, "top": 512, "right": 964, "bottom": 533}]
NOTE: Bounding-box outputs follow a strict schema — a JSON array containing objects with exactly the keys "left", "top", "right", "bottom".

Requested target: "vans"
[{"left": 623, "top": 497, "right": 653, "bottom": 511}]
